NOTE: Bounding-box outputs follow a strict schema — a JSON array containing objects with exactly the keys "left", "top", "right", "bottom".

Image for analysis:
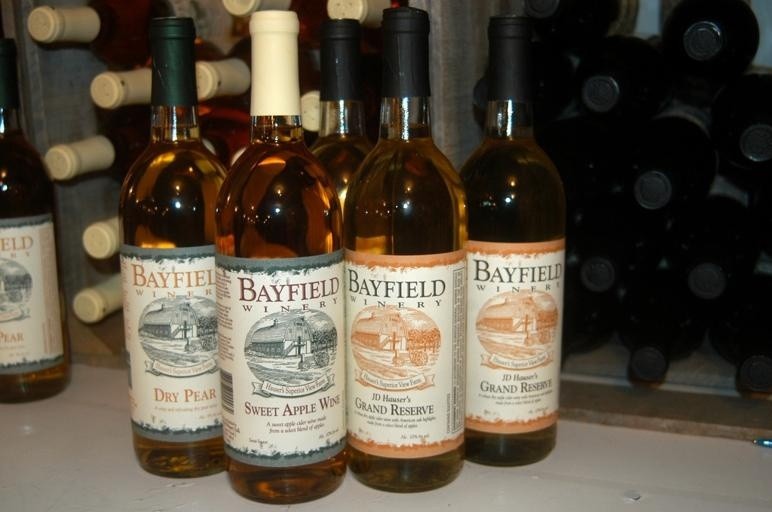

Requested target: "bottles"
[
  {"left": 216, "top": 8, "right": 350, "bottom": 503},
  {"left": 463, "top": 9, "right": 569, "bottom": 466},
  {"left": 468, "top": 0, "right": 772, "bottom": 398},
  {"left": 1, "top": 32, "right": 71, "bottom": 405},
  {"left": 313, "top": 18, "right": 370, "bottom": 195},
  {"left": 116, "top": 19, "right": 227, "bottom": 479},
  {"left": 26, "top": 0, "right": 392, "bottom": 322},
  {"left": 349, "top": 9, "right": 465, "bottom": 499}
]
